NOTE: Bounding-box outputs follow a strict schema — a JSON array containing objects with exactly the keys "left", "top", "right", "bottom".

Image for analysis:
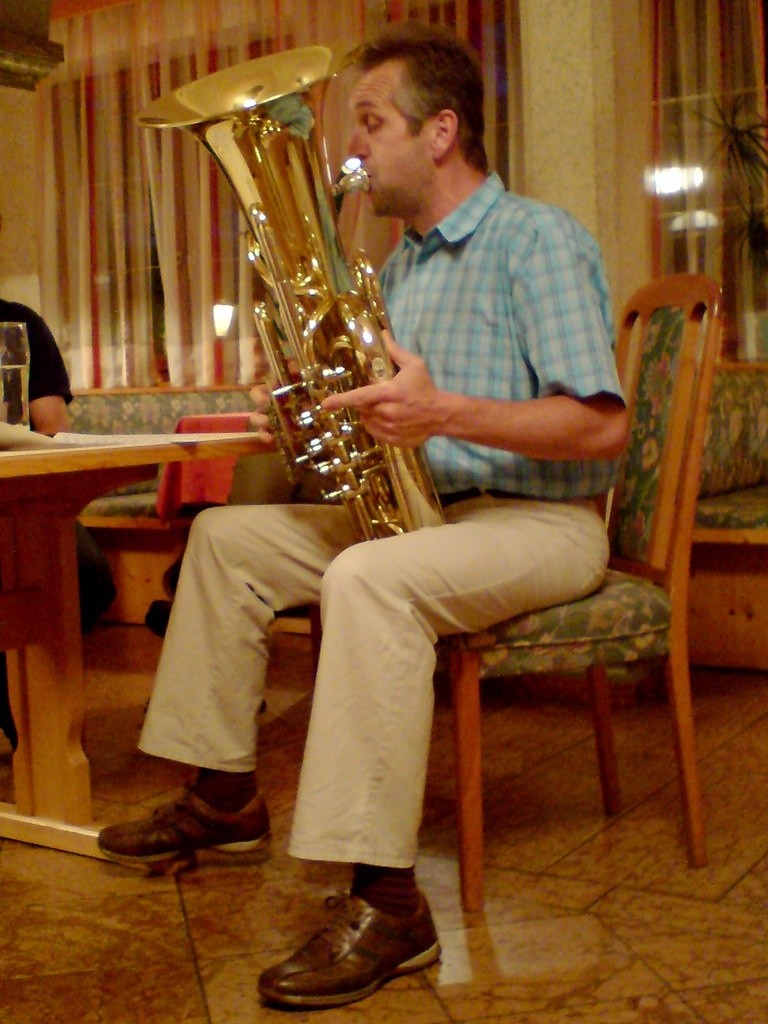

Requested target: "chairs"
[{"left": 311, "top": 272, "right": 725, "bottom": 918}]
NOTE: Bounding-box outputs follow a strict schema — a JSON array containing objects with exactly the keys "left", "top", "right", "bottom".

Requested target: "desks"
[{"left": 0, "top": 432, "right": 274, "bottom": 862}]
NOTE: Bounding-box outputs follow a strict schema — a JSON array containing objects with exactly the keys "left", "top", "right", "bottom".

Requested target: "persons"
[
  {"left": 0, "top": 301, "right": 117, "bottom": 758},
  {"left": 97, "top": 27, "right": 630, "bottom": 1005}
]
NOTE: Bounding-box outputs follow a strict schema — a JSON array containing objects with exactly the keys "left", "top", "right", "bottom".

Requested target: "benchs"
[
  {"left": 59, "top": 386, "right": 258, "bottom": 626},
  {"left": 690, "top": 359, "right": 768, "bottom": 671}
]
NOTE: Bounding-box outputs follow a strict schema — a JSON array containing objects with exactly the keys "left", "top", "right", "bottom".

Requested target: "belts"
[{"left": 432, "top": 487, "right": 529, "bottom": 508}]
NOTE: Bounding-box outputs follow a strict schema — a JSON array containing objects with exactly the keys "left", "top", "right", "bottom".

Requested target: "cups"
[{"left": 0, "top": 322, "right": 31, "bottom": 432}]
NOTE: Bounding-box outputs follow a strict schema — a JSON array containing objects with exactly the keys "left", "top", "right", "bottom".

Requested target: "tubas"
[{"left": 134, "top": 45, "right": 450, "bottom": 545}]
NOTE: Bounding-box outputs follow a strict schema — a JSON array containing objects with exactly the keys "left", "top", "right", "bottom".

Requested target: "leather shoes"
[
  {"left": 258, "top": 893, "right": 442, "bottom": 1006},
  {"left": 98, "top": 792, "right": 271, "bottom": 863}
]
